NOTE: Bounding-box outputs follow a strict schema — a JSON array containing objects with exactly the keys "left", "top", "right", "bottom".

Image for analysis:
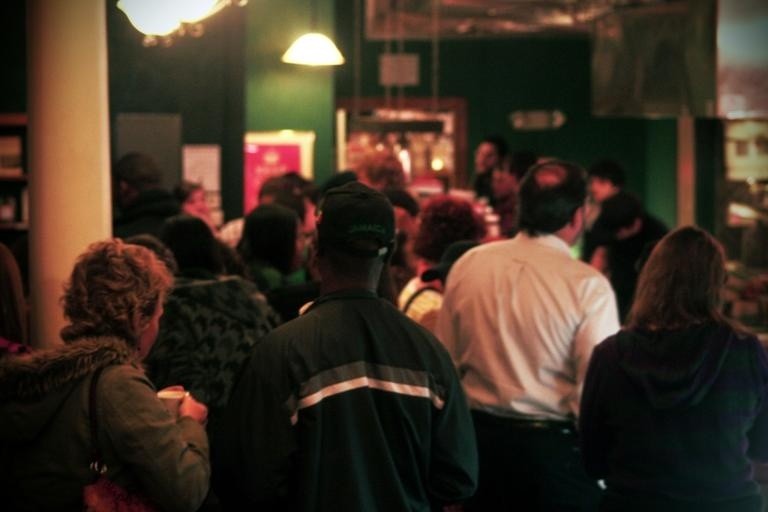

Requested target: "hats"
[{"left": 316, "top": 181, "right": 395, "bottom": 258}]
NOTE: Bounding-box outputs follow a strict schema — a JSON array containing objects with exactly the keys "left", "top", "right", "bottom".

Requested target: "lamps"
[
  {"left": 279, "top": 1, "right": 346, "bottom": 67},
  {"left": 116, "top": 0, "right": 248, "bottom": 49}
]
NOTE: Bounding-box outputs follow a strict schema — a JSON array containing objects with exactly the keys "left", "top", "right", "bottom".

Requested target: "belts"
[{"left": 509, "top": 421, "right": 573, "bottom": 433}]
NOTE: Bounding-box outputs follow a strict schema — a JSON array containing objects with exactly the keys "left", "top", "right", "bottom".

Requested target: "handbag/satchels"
[{"left": 84, "top": 476, "right": 148, "bottom": 512}]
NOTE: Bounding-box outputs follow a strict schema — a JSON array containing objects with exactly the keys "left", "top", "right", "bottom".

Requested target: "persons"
[
  {"left": 140, "top": 216, "right": 281, "bottom": 417},
  {"left": 581, "top": 225, "right": 768, "bottom": 512},
  {"left": 435, "top": 160, "right": 621, "bottom": 512},
  {"left": 226, "top": 182, "right": 479, "bottom": 512},
  {"left": 1, "top": 238, "right": 211, "bottom": 512},
  {"left": 111, "top": 133, "right": 646, "bottom": 326}
]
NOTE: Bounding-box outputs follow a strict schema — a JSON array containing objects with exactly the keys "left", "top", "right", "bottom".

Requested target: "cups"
[{"left": 157, "top": 391, "right": 190, "bottom": 418}]
